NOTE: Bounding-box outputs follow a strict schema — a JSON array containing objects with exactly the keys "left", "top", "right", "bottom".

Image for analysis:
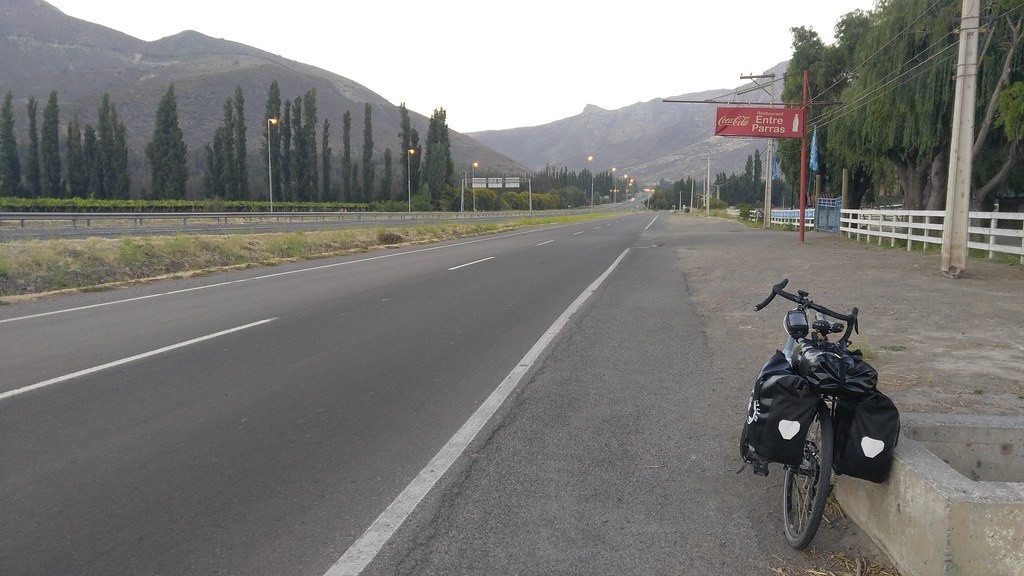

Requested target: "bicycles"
[{"left": 754, "top": 279, "right": 859, "bottom": 550}]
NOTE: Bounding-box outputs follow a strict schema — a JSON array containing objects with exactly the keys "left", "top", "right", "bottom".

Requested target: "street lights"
[
  {"left": 473, "top": 163, "right": 477, "bottom": 212},
  {"left": 610, "top": 190, "right": 612, "bottom": 205},
  {"left": 268, "top": 119, "right": 276, "bottom": 212},
  {"left": 624, "top": 175, "right": 628, "bottom": 210},
  {"left": 408, "top": 149, "right": 414, "bottom": 213},
  {"left": 612, "top": 168, "right": 617, "bottom": 212},
  {"left": 588, "top": 156, "right": 593, "bottom": 209},
  {"left": 645, "top": 189, "right": 654, "bottom": 210}
]
